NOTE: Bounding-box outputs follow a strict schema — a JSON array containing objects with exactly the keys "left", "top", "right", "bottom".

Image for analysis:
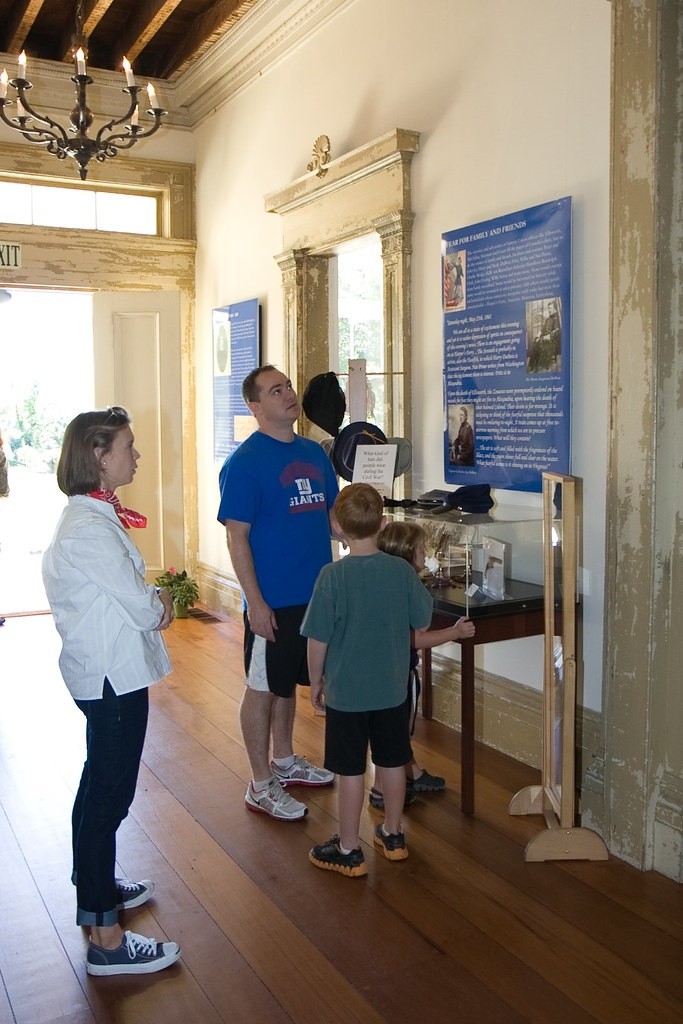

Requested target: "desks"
[{"left": 424, "top": 575, "right": 583, "bottom": 815}]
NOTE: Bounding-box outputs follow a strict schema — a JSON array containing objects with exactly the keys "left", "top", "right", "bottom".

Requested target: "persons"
[
  {"left": 300, "top": 483, "right": 433, "bottom": 877},
  {"left": 368, "top": 520, "right": 475, "bottom": 810},
  {"left": 42, "top": 407, "right": 181, "bottom": 977},
  {"left": 449, "top": 257, "right": 467, "bottom": 302},
  {"left": 449, "top": 407, "right": 476, "bottom": 462},
  {"left": 215, "top": 364, "right": 387, "bottom": 823},
  {"left": 527, "top": 301, "right": 560, "bottom": 372}
]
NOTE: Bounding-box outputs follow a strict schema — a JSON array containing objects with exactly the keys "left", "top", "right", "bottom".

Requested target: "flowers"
[{"left": 154, "top": 567, "right": 199, "bottom": 608}]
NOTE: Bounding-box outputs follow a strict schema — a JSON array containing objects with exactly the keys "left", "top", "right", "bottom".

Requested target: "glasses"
[{"left": 96, "top": 407, "right": 128, "bottom": 435}]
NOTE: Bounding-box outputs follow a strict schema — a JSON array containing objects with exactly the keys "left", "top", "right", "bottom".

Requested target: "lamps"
[{"left": 0, "top": 0, "right": 169, "bottom": 181}]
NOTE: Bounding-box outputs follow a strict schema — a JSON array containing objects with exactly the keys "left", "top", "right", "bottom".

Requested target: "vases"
[{"left": 173, "top": 603, "right": 190, "bottom": 618}]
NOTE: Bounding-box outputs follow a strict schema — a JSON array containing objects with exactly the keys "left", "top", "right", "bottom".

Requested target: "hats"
[
  {"left": 302, "top": 372, "right": 346, "bottom": 437},
  {"left": 332, "top": 422, "right": 388, "bottom": 482},
  {"left": 388, "top": 437, "right": 413, "bottom": 477},
  {"left": 320, "top": 440, "right": 340, "bottom": 482}
]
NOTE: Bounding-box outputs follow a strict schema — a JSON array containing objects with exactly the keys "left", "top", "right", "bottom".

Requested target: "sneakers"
[
  {"left": 308, "top": 834, "right": 369, "bottom": 877},
  {"left": 269, "top": 755, "right": 335, "bottom": 787},
  {"left": 368, "top": 787, "right": 416, "bottom": 808},
  {"left": 413, "top": 769, "right": 445, "bottom": 791},
  {"left": 244, "top": 780, "right": 309, "bottom": 821},
  {"left": 374, "top": 822, "right": 409, "bottom": 861},
  {"left": 114, "top": 878, "right": 156, "bottom": 911},
  {"left": 85, "top": 930, "right": 183, "bottom": 976}
]
